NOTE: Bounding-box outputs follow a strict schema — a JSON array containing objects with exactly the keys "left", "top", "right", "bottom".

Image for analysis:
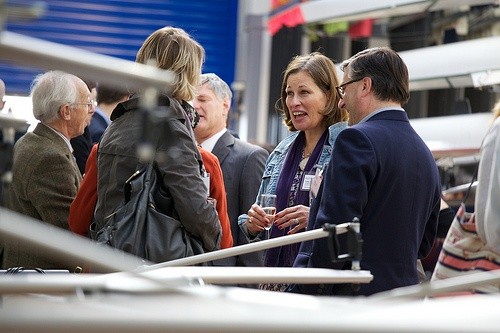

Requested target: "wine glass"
[{"left": 259, "top": 193, "right": 277, "bottom": 240}]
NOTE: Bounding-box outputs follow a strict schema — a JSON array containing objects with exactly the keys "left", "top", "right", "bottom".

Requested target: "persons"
[
  {"left": 1, "top": 27, "right": 271, "bottom": 293},
  {"left": 475, "top": 100, "right": 499, "bottom": 297},
  {"left": 238, "top": 47, "right": 475, "bottom": 297}
]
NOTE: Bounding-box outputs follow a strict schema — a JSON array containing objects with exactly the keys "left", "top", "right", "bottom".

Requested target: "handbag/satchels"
[
  {"left": 89, "top": 118, "right": 216, "bottom": 267},
  {"left": 422, "top": 158, "right": 500, "bottom": 302}
]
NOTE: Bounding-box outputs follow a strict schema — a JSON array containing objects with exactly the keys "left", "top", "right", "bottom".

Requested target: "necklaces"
[{"left": 306, "top": 128, "right": 326, "bottom": 153}]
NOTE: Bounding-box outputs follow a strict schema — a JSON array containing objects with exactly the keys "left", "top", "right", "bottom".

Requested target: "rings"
[
  {"left": 249, "top": 216, "right": 252, "bottom": 223},
  {"left": 294, "top": 219, "right": 298, "bottom": 223}
]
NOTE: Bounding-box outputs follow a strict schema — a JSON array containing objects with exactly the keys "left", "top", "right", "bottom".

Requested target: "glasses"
[
  {"left": 336, "top": 76, "right": 364, "bottom": 99},
  {"left": 69, "top": 99, "right": 92, "bottom": 109}
]
